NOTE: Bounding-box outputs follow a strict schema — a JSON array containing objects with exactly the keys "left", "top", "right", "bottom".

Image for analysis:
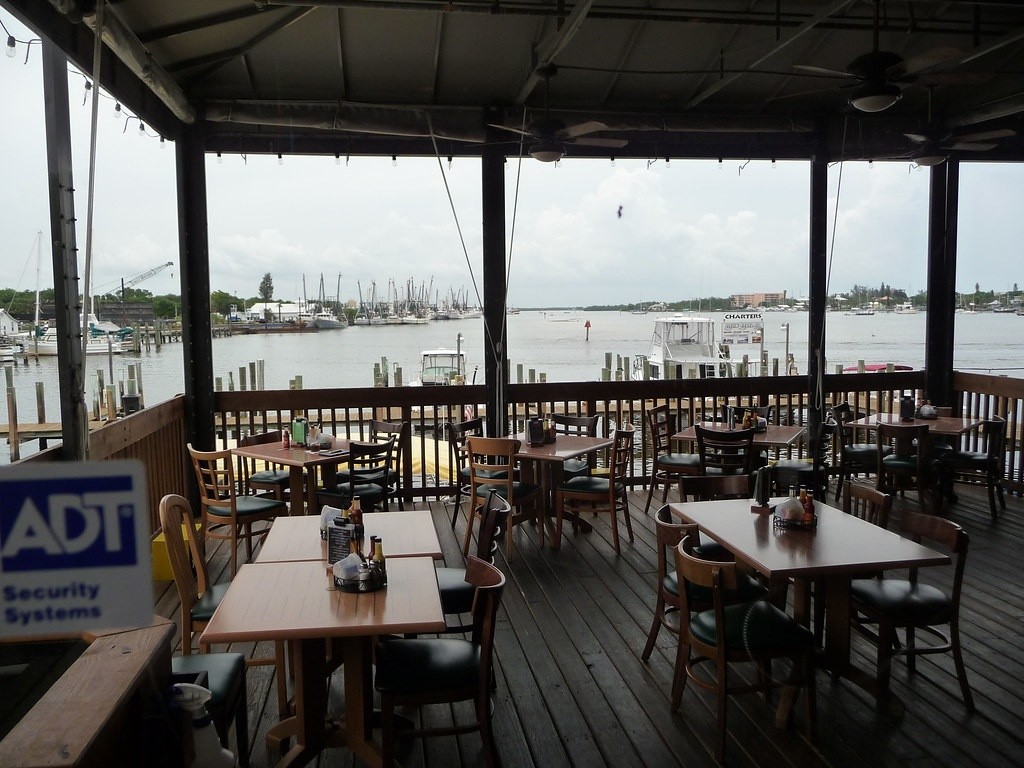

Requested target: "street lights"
[{"left": 781, "top": 322, "right": 789, "bottom": 375}]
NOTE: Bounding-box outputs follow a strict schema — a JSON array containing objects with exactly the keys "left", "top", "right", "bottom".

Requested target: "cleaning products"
[{"left": 171, "top": 683, "right": 241, "bottom": 768}]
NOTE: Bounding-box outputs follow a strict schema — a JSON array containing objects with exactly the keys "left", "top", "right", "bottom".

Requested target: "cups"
[{"left": 309, "top": 435, "right": 320, "bottom": 452}]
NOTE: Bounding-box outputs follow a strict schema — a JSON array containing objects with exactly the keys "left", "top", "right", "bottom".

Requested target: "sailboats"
[
  {"left": 633, "top": 299, "right": 647, "bottom": 314},
  {"left": 297, "top": 272, "right": 350, "bottom": 329},
  {"left": 354, "top": 275, "right": 482, "bottom": 325},
  {"left": 28, "top": 229, "right": 133, "bottom": 356}
]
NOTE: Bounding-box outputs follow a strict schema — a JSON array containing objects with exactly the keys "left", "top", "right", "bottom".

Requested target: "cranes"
[{"left": 89, "top": 262, "right": 174, "bottom": 326}]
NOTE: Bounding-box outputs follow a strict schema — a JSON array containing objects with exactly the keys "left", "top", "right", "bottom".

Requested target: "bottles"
[
  {"left": 544, "top": 413, "right": 556, "bottom": 441},
  {"left": 375, "top": 560, "right": 384, "bottom": 581},
  {"left": 788, "top": 486, "right": 797, "bottom": 499},
  {"left": 284, "top": 425, "right": 290, "bottom": 450},
  {"left": 799, "top": 485, "right": 806, "bottom": 504},
  {"left": 745, "top": 410, "right": 751, "bottom": 429},
  {"left": 341, "top": 505, "right": 350, "bottom": 524},
  {"left": 369, "top": 535, "right": 378, "bottom": 560},
  {"left": 351, "top": 496, "right": 363, "bottom": 524},
  {"left": 359, "top": 563, "right": 369, "bottom": 591},
  {"left": 804, "top": 489, "right": 814, "bottom": 529},
  {"left": 917, "top": 397, "right": 931, "bottom": 406},
  {"left": 742, "top": 409, "right": 748, "bottom": 429},
  {"left": 332, "top": 552, "right": 367, "bottom": 579},
  {"left": 372, "top": 537, "right": 385, "bottom": 567},
  {"left": 753, "top": 410, "right": 758, "bottom": 427},
  {"left": 368, "top": 561, "right": 377, "bottom": 587}
]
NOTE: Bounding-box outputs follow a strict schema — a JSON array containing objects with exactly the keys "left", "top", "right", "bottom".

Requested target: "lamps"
[
  {"left": 849, "top": 82, "right": 903, "bottom": 114},
  {"left": 526, "top": 140, "right": 567, "bottom": 161},
  {"left": 911, "top": 147, "right": 949, "bottom": 166}
]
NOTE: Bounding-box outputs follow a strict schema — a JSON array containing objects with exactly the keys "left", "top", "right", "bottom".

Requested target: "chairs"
[{"left": 160, "top": 402, "right": 1007, "bottom": 748}]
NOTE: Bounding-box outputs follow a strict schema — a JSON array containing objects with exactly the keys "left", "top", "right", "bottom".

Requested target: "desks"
[
  {"left": 845, "top": 414, "right": 983, "bottom": 455},
  {"left": 670, "top": 422, "right": 807, "bottom": 469},
  {"left": 231, "top": 437, "right": 350, "bottom": 517},
  {"left": 200, "top": 557, "right": 448, "bottom": 767},
  {"left": 671, "top": 496, "right": 952, "bottom": 742},
  {"left": 496, "top": 430, "right": 615, "bottom": 550},
  {"left": 254, "top": 509, "right": 444, "bottom": 583}
]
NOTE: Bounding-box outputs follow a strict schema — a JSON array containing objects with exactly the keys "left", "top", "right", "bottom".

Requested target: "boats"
[
  {"left": 959, "top": 311, "right": 977, "bottom": 314},
  {"left": 412, "top": 348, "right": 466, "bottom": 388},
  {"left": 993, "top": 307, "right": 1016, "bottom": 312},
  {"left": 898, "top": 309, "right": 916, "bottom": 313},
  {"left": 631, "top": 314, "right": 734, "bottom": 381}
]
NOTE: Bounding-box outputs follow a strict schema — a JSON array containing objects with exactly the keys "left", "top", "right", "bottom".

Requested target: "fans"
[
  {"left": 763, "top": 1, "right": 957, "bottom": 106},
  {"left": 486, "top": 63, "right": 628, "bottom": 159},
  {"left": 898, "top": 84, "right": 1015, "bottom": 166}
]
